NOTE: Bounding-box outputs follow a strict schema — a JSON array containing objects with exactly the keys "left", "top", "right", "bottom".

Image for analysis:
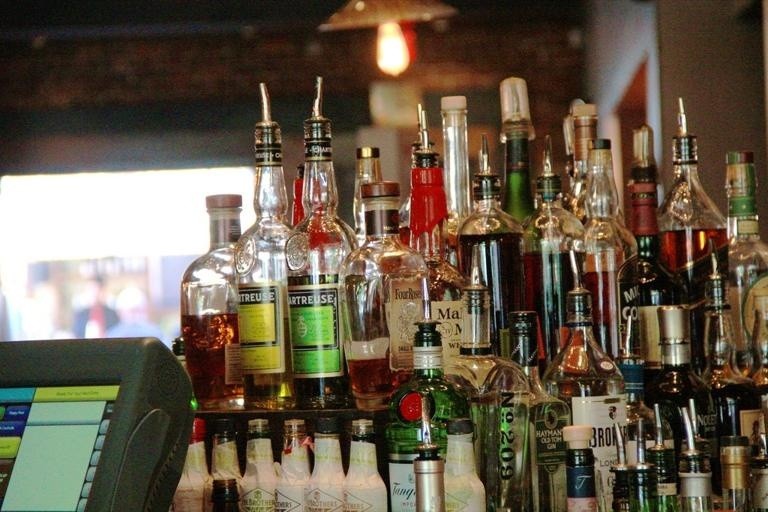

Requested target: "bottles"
[{"left": 169, "top": 73, "right": 768, "bottom": 511}]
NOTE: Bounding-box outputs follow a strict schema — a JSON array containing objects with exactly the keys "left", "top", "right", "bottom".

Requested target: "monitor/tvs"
[{"left": 0, "top": 339, "right": 195, "bottom": 512}]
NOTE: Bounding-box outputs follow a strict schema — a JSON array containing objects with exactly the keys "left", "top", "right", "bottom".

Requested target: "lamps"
[{"left": 311, "top": 1, "right": 462, "bottom": 76}]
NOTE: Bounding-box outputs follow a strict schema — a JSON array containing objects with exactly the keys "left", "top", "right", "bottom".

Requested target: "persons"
[{"left": 22, "top": 274, "right": 163, "bottom": 341}]
